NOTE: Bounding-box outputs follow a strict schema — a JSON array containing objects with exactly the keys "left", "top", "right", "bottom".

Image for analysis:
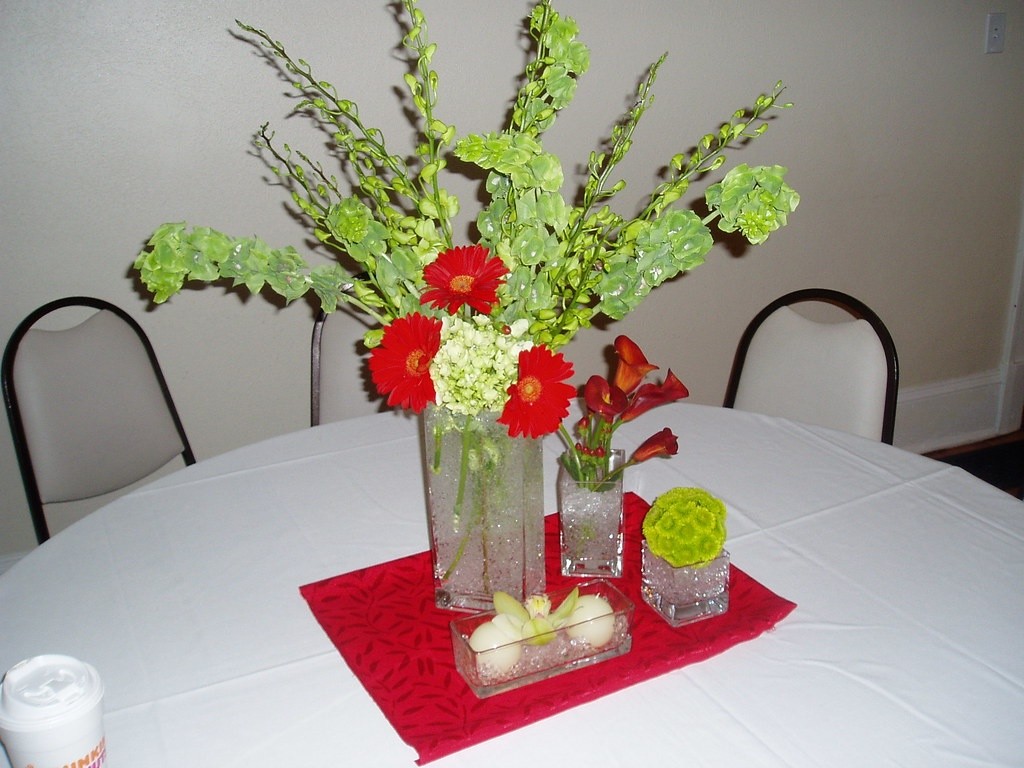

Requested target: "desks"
[{"left": 0, "top": 398, "right": 1024, "bottom": 768}]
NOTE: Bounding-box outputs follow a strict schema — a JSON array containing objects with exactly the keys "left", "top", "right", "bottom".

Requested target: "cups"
[{"left": 0, "top": 654, "right": 106, "bottom": 768}]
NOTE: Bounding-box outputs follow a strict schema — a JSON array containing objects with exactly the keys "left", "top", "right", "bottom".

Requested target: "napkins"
[{"left": 297, "top": 492, "right": 796, "bottom": 767}]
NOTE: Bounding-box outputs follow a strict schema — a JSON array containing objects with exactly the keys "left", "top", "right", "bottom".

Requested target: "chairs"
[
  {"left": 0, "top": 296, "right": 197, "bottom": 546},
  {"left": 721, "top": 289, "right": 899, "bottom": 445},
  {"left": 310, "top": 272, "right": 402, "bottom": 428}
]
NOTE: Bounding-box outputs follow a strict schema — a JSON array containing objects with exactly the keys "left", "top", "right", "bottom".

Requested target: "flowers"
[
  {"left": 496, "top": 335, "right": 690, "bottom": 557},
  {"left": 642, "top": 486, "right": 727, "bottom": 569},
  {"left": 130, "top": 0, "right": 802, "bottom": 586}
]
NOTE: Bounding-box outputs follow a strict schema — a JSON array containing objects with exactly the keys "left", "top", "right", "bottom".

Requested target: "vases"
[
  {"left": 641, "top": 540, "right": 729, "bottom": 626},
  {"left": 421, "top": 402, "right": 545, "bottom": 612},
  {"left": 558, "top": 448, "right": 623, "bottom": 577}
]
